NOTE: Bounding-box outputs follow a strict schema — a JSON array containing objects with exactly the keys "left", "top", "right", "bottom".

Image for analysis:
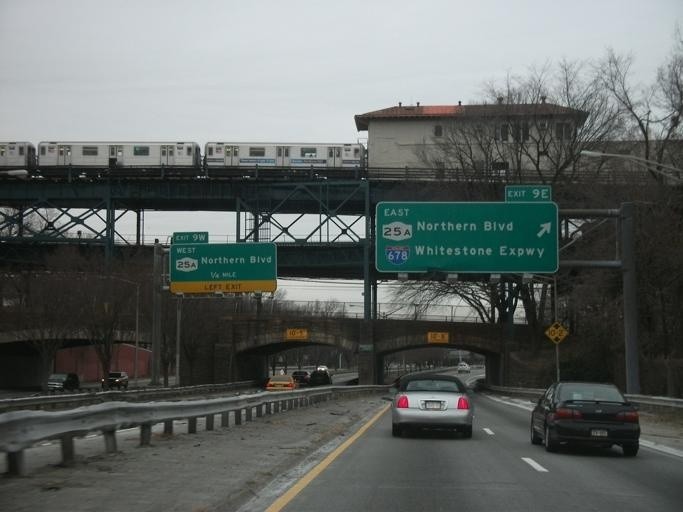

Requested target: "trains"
[{"left": 0, "top": 140, "right": 366, "bottom": 180}]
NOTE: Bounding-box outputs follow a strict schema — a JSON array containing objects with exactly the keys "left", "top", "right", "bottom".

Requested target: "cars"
[
  {"left": 457, "top": 362, "right": 470, "bottom": 373},
  {"left": 530, "top": 381, "right": 639, "bottom": 457},
  {"left": 101, "top": 372, "right": 128, "bottom": 390},
  {"left": 41, "top": 372, "right": 79, "bottom": 392},
  {"left": 391, "top": 374, "right": 472, "bottom": 437},
  {"left": 265, "top": 365, "right": 333, "bottom": 391}
]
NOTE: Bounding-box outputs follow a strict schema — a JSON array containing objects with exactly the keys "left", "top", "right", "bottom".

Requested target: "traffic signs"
[
  {"left": 504, "top": 184, "right": 551, "bottom": 202},
  {"left": 373, "top": 201, "right": 559, "bottom": 274},
  {"left": 168, "top": 242, "right": 277, "bottom": 293},
  {"left": 173, "top": 231, "right": 208, "bottom": 242}
]
